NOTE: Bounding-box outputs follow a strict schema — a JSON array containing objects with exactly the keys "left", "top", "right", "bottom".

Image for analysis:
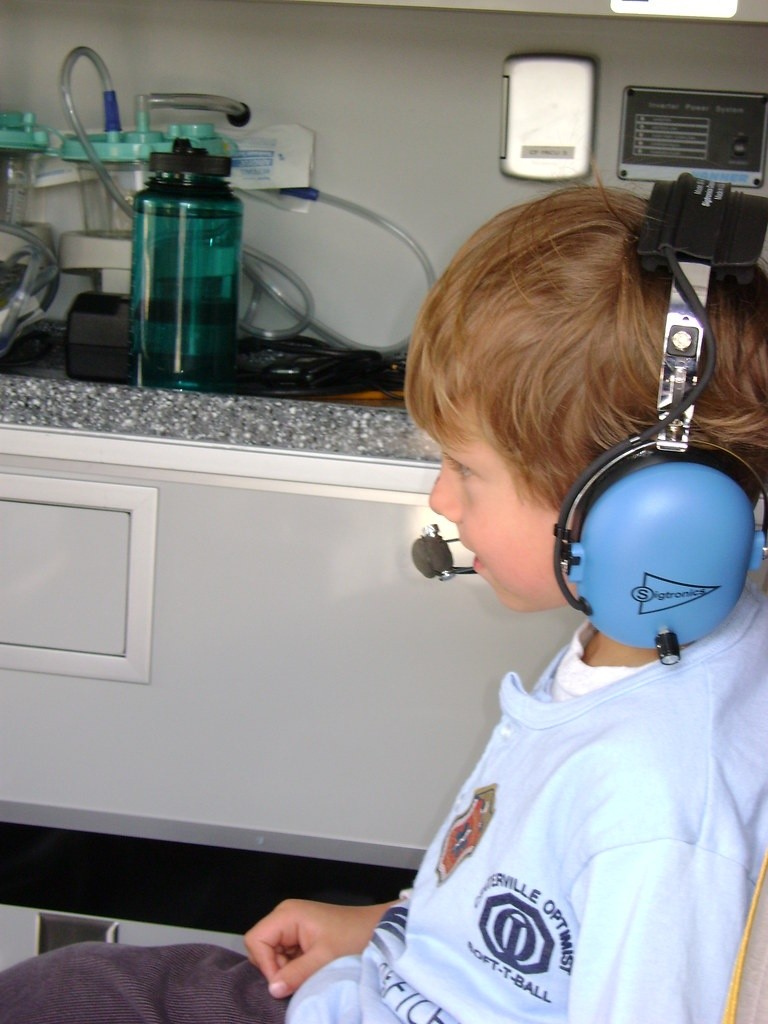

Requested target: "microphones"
[{"left": 408, "top": 522, "right": 479, "bottom": 581}]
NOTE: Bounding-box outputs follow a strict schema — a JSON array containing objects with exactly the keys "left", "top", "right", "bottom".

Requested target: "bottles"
[{"left": 127, "top": 141, "right": 245, "bottom": 390}]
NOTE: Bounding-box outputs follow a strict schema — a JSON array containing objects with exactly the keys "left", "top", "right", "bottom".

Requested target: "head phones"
[{"left": 555, "top": 172, "right": 767, "bottom": 663}]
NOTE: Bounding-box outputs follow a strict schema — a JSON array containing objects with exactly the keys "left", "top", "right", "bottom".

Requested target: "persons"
[{"left": 0, "top": 186, "right": 768, "bottom": 1023}]
